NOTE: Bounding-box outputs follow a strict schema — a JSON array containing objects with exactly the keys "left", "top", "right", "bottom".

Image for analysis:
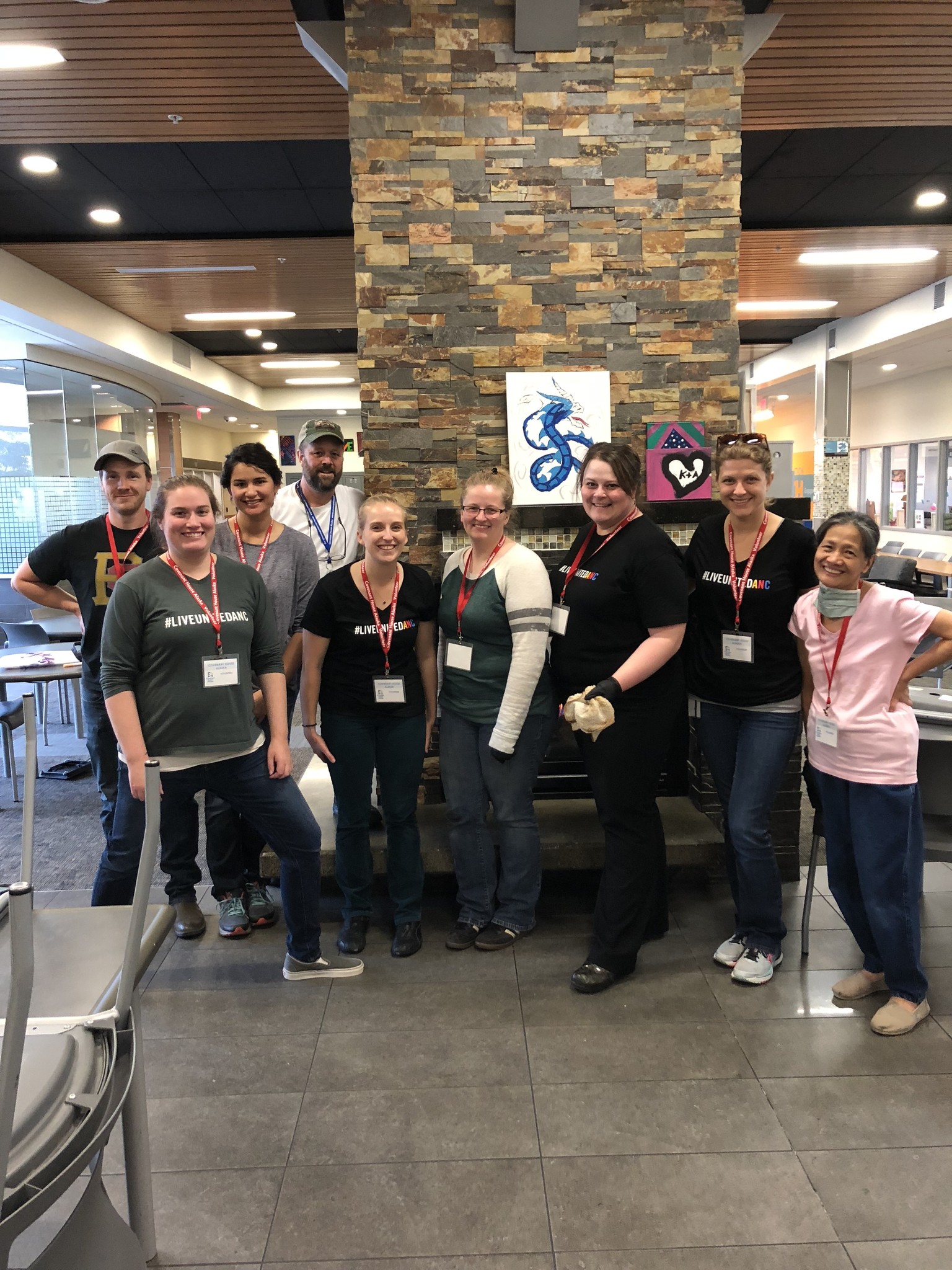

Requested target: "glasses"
[
  {"left": 715, "top": 432, "right": 771, "bottom": 453},
  {"left": 308, "top": 518, "right": 347, "bottom": 561},
  {"left": 461, "top": 505, "right": 506, "bottom": 518}
]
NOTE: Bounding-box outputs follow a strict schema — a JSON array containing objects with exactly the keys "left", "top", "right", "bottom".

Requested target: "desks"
[
  {"left": 876, "top": 551, "right": 952, "bottom": 597},
  {"left": 0, "top": 642, "right": 84, "bottom": 777},
  {"left": 2, "top": 904, "right": 178, "bottom": 1260},
  {"left": 17, "top": 614, "right": 82, "bottom": 641}
]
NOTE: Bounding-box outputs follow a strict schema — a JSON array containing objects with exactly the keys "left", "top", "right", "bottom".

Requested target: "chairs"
[
  {"left": 0, "top": 623, "right": 64, "bottom": 746},
  {"left": 0, "top": 696, "right": 24, "bottom": 802},
  {"left": 899, "top": 548, "right": 922, "bottom": 557},
  {"left": 880, "top": 541, "right": 904, "bottom": 554},
  {"left": 30, "top": 606, "right": 74, "bottom": 619},
  {"left": 868, "top": 556, "right": 917, "bottom": 578},
  {"left": 0, "top": 691, "right": 147, "bottom": 1270},
  {"left": 921, "top": 551, "right": 947, "bottom": 560}
]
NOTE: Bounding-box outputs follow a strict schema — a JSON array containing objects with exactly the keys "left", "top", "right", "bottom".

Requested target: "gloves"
[
  {"left": 491, "top": 748, "right": 516, "bottom": 765},
  {"left": 433, "top": 717, "right": 441, "bottom": 727},
  {"left": 584, "top": 676, "right": 624, "bottom": 707}
]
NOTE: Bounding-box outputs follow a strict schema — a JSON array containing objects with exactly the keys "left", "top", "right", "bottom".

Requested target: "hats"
[
  {"left": 94, "top": 440, "right": 151, "bottom": 471},
  {"left": 299, "top": 419, "right": 345, "bottom": 449}
]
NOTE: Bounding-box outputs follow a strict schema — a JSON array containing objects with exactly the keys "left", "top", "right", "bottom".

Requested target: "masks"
[{"left": 813, "top": 582, "right": 861, "bottom": 617}]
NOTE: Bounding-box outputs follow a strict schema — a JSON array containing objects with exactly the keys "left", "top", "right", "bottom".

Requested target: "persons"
[
  {"left": 91, "top": 474, "right": 364, "bottom": 980},
  {"left": 550, "top": 442, "right": 698, "bottom": 995},
  {"left": 684, "top": 435, "right": 819, "bottom": 985},
  {"left": 789, "top": 512, "right": 952, "bottom": 1035},
  {"left": 210, "top": 443, "right": 320, "bottom": 937},
  {"left": 437, "top": 467, "right": 554, "bottom": 949},
  {"left": 300, "top": 493, "right": 438, "bottom": 959},
  {"left": 13, "top": 441, "right": 169, "bottom": 909},
  {"left": 268, "top": 418, "right": 381, "bottom": 825}
]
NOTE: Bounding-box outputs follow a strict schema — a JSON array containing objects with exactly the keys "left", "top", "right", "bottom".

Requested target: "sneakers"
[
  {"left": 474, "top": 922, "right": 529, "bottom": 950},
  {"left": 215, "top": 891, "right": 252, "bottom": 936},
  {"left": 246, "top": 876, "right": 275, "bottom": 925},
  {"left": 446, "top": 920, "right": 487, "bottom": 949},
  {"left": 731, "top": 946, "right": 783, "bottom": 984},
  {"left": 390, "top": 920, "right": 422, "bottom": 956},
  {"left": 713, "top": 933, "right": 748, "bottom": 967},
  {"left": 336, "top": 915, "right": 369, "bottom": 954}
]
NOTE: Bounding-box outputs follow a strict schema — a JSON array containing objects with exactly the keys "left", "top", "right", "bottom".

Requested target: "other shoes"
[
  {"left": 168, "top": 901, "right": 206, "bottom": 937},
  {"left": 870, "top": 997, "right": 931, "bottom": 1034},
  {"left": 283, "top": 950, "right": 365, "bottom": 980},
  {"left": 570, "top": 960, "right": 614, "bottom": 994},
  {"left": 831, "top": 970, "right": 889, "bottom": 1000}
]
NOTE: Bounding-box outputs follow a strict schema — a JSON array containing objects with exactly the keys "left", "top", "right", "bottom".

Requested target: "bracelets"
[{"left": 302, "top": 723, "right": 317, "bottom": 727}]
